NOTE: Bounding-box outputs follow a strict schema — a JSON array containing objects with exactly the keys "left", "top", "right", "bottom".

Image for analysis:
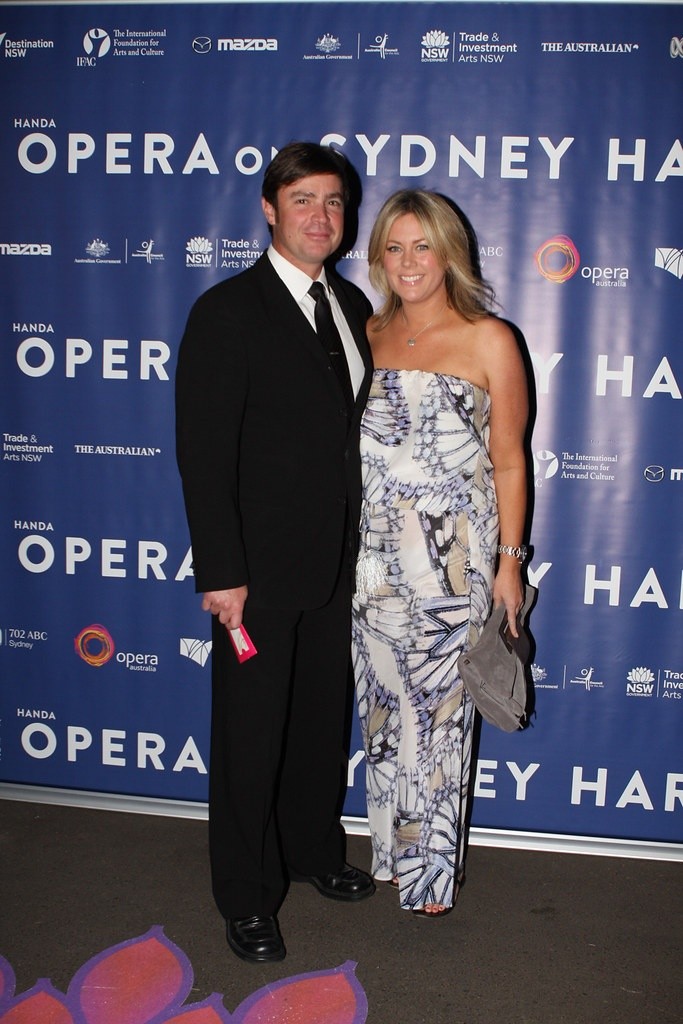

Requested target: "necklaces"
[{"left": 401, "top": 303, "right": 447, "bottom": 347}]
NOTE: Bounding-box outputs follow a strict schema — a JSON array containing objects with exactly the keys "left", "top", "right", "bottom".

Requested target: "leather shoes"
[
  {"left": 226, "top": 913, "right": 287, "bottom": 965},
  {"left": 310, "top": 863, "right": 377, "bottom": 902}
]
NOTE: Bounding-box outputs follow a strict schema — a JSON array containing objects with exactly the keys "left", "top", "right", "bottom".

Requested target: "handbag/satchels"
[{"left": 457, "top": 580, "right": 536, "bottom": 733}]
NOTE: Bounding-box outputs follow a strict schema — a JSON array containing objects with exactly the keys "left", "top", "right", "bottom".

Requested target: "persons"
[
  {"left": 174, "top": 144, "right": 381, "bottom": 967},
  {"left": 351, "top": 186, "right": 534, "bottom": 920}
]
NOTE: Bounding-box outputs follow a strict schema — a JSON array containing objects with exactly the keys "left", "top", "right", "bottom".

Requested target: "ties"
[{"left": 306, "top": 283, "right": 357, "bottom": 410}]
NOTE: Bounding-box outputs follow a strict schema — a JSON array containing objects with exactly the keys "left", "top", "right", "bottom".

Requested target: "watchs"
[{"left": 498, "top": 544, "right": 534, "bottom": 561}]
